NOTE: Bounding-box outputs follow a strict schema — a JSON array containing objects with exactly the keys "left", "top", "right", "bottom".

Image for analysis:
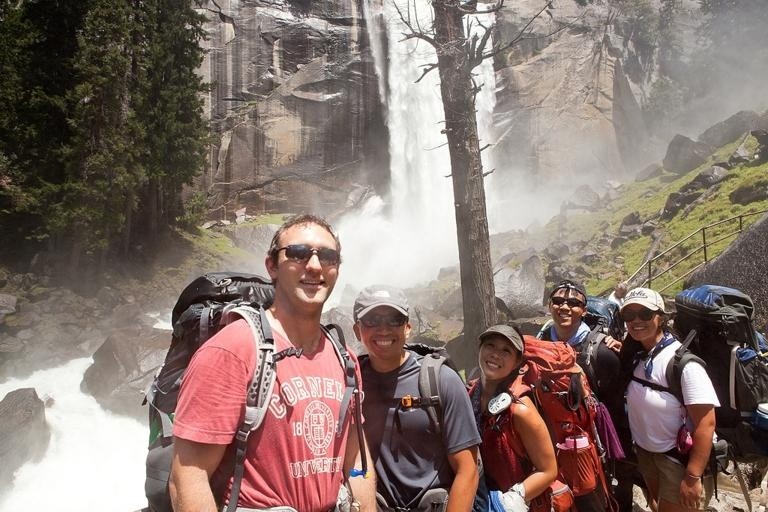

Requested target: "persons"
[
  {"left": 353, "top": 282, "right": 482, "bottom": 512},
  {"left": 604, "top": 287, "right": 722, "bottom": 512},
  {"left": 168, "top": 213, "right": 365, "bottom": 512},
  {"left": 536, "top": 279, "right": 625, "bottom": 512},
  {"left": 466, "top": 321, "right": 559, "bottom": 512}
]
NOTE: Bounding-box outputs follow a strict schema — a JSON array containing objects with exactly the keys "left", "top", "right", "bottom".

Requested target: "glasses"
[
  {"left": 621, "top": 310, "right": 653, "bottom": 321},
  {"left": 276, "top": 244, "right": 339, "bottom": 266},
  {"left": 363, "top": 312, "right": 405, "bottom": 327}
]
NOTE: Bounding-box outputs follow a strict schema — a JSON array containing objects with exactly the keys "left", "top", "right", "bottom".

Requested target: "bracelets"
[{"left": 686, "top": 473, "right": 700, "bottom": 482}]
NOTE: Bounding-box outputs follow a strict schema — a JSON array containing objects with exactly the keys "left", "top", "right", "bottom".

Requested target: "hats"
[
  {"left": 621, "top": 288, "right": 665, "bottom": 314},
  {"left": 479, "top": 323, "right": 524, "bottom": 357},
  {"left": 353, "top": 286, "right": 410, "bottom": 318},
  {"left": 551, "top": 280, "right": 587, "bottom": 306}
]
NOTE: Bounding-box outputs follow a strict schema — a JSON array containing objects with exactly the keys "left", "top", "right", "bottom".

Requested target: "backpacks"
[
  {"left": 144, "top": 272, "right": 359, "bottom": 512},
  {"left": 537, "top": 294, "right": 623, "bottom": 394},
  {"left": 665, "top": 284, "right": 768, "bottom": 464},
  {"left": 463, "top": 334, "right": 610, "bottom": 511}
]
